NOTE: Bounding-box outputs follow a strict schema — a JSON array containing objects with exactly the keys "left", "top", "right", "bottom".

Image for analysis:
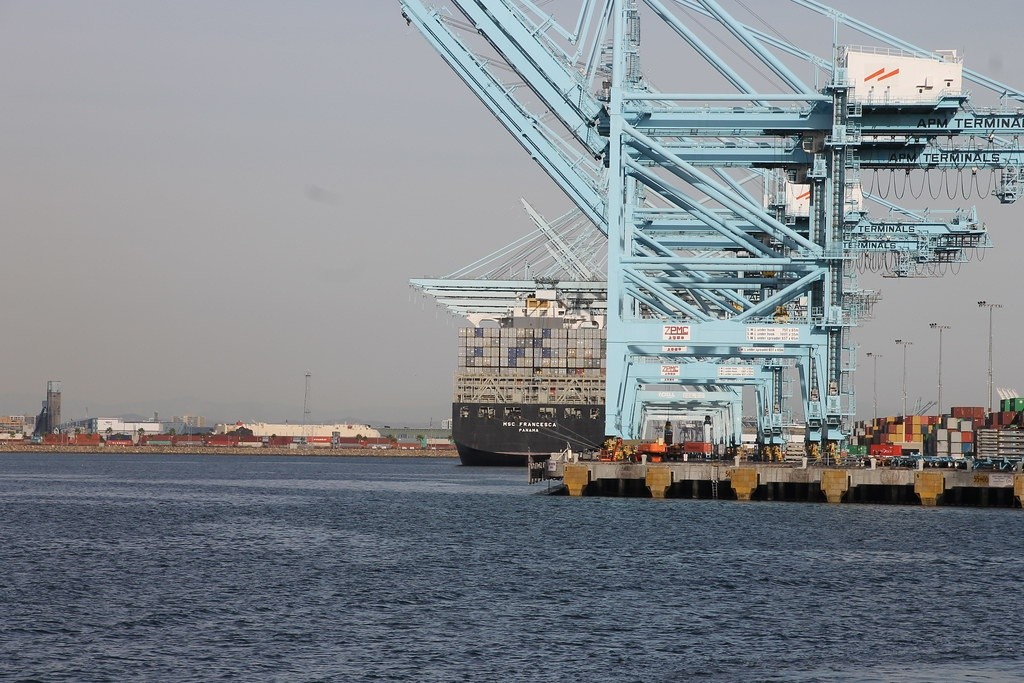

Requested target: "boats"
[{"left": 449, "top": 314, "right": 606, "bottom": 467}]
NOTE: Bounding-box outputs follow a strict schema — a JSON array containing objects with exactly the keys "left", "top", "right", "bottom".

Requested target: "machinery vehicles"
[{"left": 599, "top": 437, "right": 637, "bottom": 461}]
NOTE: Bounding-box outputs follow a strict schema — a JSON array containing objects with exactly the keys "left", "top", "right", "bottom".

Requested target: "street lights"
[
  {"left": 867, "top": 352, "right": 883, "bottom": 427},
  {"left": 977, "top": 300, "right": 1004, "bottom": 411},
  {"left": 894, "top": 339, "right": 914, "bottom": 419},
  {"left": 928, "top": 322, "right": 951, "bottom": 416}
]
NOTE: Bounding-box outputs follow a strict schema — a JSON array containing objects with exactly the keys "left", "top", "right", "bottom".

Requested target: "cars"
[{"left": 867, "top": 454, "right": 1018, "bottom": 471}]
